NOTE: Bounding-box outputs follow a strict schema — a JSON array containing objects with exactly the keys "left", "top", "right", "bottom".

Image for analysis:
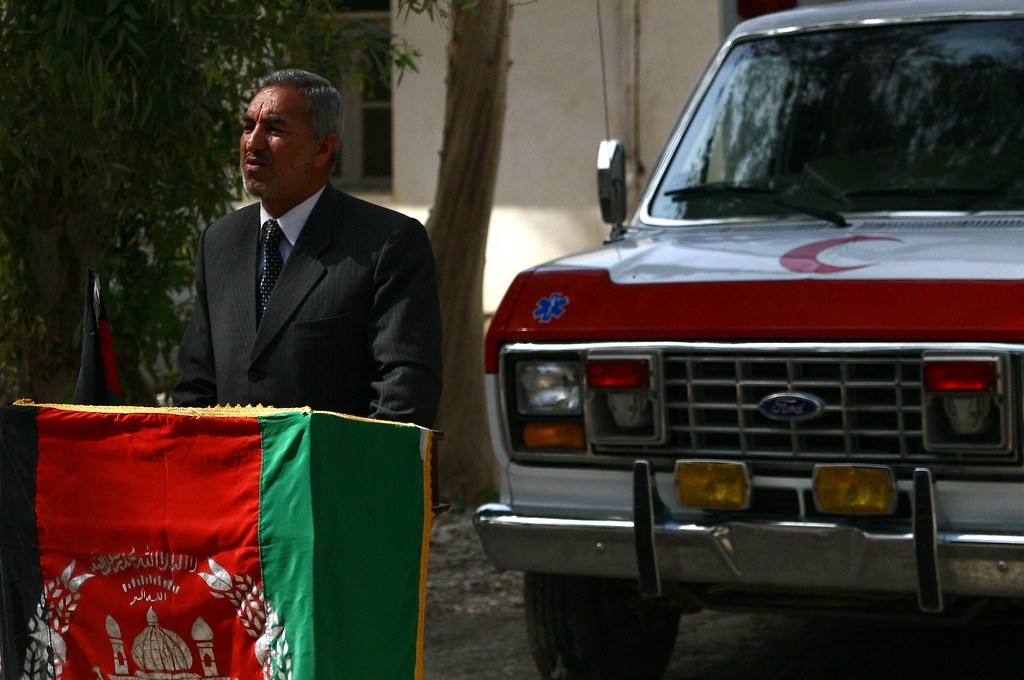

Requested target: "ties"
[{"left": 253, "top": 219, "right": 285, "bottom": 333}]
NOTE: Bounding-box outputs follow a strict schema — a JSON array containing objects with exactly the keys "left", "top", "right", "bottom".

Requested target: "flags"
[
  {"left": 72, "top": 271, "right": 125, "bottom": 406},
  {"left": 0, "top": 404, "right": 435, "bottom": 680}
]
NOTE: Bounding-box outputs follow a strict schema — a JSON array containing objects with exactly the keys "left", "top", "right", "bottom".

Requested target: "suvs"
[{"left": 473, "top": 0, "right": 1024, "bottom": 680}]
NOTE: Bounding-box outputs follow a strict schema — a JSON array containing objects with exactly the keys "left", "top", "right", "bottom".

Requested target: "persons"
[{"left": 173, "top": 70, "right": 445, "bottom": 429}]
